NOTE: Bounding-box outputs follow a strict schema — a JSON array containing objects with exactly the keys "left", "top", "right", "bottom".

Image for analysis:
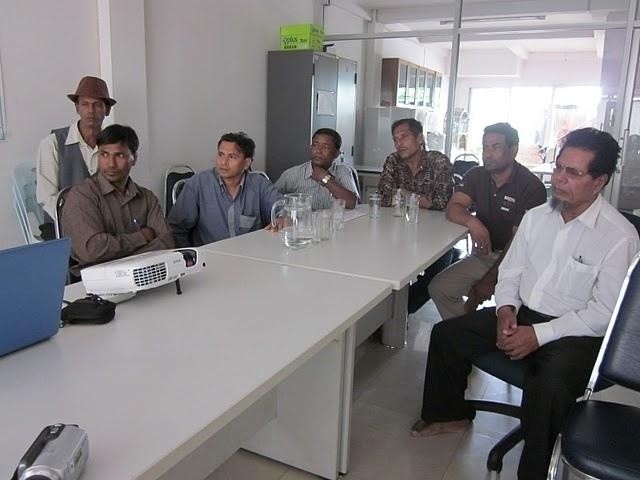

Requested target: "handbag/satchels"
[{"left": 59, "top": 297, "right": 116, "bottom": 328}]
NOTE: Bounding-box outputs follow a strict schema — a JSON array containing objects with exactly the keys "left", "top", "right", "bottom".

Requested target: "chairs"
[
  {"left": 456, "top": 207, "right": 640, "bottom": 480},
  {"left": 346, "top": 166, "right": 360, "bottom": 194},
  {"left": 51, "top": 183, "right": 83, "bottom": 286},
  {"left": 161, "top": 160, "right": 197, "bottom": 221},
  {"left": 451, "top": 150, "right": 478, "bottom": 175},
  {"left": 171, "top": 177, "right": 191, "bottom": 206},
  {"left": 9, "top": 159, "right": 48, "bottom": 243},
  {"left": 248, "top": 169, "right": 270, "bottom": 181},
  {"left": 540, "top": 243, "right": 640, "bottom": 480}
]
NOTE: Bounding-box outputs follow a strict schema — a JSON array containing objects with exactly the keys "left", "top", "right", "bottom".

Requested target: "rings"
[{"left": 480, "top": 246, "right": 488, "bottom": 249}]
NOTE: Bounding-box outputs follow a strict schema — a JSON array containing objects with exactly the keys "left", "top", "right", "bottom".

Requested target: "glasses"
[{"left": 552, "top": 163, "right": 592, "bottom": 178}]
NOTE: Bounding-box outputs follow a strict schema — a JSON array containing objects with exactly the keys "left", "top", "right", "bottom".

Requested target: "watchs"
[{"left": 319, "top": 175, "right": 331, "bottom": 187}]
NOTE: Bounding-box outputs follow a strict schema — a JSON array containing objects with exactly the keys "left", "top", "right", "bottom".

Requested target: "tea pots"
[{"left": 270, "top": 189, "right": 314, "bottom": 249}]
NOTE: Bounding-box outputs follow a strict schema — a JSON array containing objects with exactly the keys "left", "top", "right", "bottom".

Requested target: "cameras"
[{"left": 10, "top": 423, "right": 89, "bottom": 480}]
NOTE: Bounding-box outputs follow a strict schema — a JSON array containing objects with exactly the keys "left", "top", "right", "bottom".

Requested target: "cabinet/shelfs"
[
  {"left": 264, "top": 45, "right": 359, "bottom": 188},
  {"left": 379, "top": 54, "right": 444, "bottom": 112}
]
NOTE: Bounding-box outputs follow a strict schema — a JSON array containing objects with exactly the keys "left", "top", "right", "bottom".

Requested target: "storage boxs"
[{"left": 279, "top": 22, "right": 328, "bottom": 51}]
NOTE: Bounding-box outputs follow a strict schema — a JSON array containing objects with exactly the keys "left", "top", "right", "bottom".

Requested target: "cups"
[
  {"left": 369, "top": 194, "right": 382, "bottom": 219},
  {"left": 315, "top": 209, "right": 331, "bottom": 242},
  {"left": 391, "top": 193, "right": 420, "bottom": 224},
  {"left": 330, "top": 199, "right": 346, "bottom": 232}
]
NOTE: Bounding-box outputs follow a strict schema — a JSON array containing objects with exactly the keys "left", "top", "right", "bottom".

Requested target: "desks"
[
  {"left": 528, "top": 160, "right": 556, "bottom": 184},
  {"left": 197, "top": 194, "right": 475, "bottom": 477},
  {"left": 1, "top": 241, "right": 394, "bottom": 480}
]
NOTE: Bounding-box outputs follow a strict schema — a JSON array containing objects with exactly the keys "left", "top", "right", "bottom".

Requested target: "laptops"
[{"left": 0, "top": 237, "right": 71, "bottom": 358}]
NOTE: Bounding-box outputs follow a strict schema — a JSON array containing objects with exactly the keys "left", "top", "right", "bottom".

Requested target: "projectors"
[{"left": 80, "top": 247, "right": 207, "bottom": 303}]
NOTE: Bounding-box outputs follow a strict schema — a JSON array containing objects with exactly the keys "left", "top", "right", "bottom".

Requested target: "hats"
[{"left": 67, "top": 77, "right": 116, "bottom": 105}]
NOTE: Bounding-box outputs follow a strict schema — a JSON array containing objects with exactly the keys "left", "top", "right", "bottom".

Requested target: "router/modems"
[{"left": 61, "top": 296, "right": 116, "bottom": 325}]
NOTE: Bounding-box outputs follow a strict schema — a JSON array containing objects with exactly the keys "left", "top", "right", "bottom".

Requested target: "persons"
[
  {"left": 165, "top": 132, "right": 291, "bottom": 248},
  {"left": 275, "top": 128, "right": 362, "bottom": 215},
  {"left": 63, "top": 125, "right": 175, "bottom": 273},
  {"left": 373, "top": 119, "right": 454, "bottom": 338},
  {"left": 427, "top": 124, "right": 546, "bottom": 320},
  {"left": 36, "top": 76, "right": 116, "bottom": 243},
  {"left": 414, "top": 128, "right": 640, "bottom": 479}
]
